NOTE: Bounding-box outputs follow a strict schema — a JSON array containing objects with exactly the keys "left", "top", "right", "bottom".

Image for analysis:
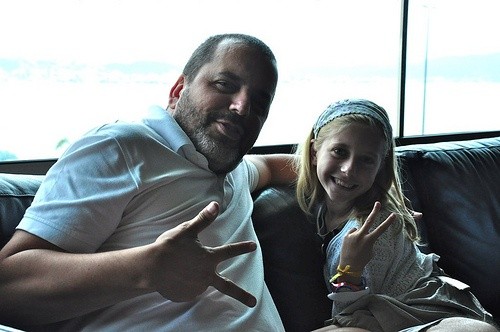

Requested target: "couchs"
[{"left": 0, "top": 137, "right": 500, "bottom": 332}]
